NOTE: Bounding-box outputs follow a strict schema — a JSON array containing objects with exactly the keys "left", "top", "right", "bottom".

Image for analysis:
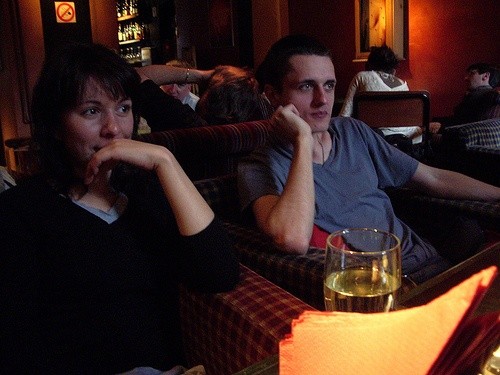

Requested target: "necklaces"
[{"left": 317, "top": 138, "right": 325, "bottom": 163}]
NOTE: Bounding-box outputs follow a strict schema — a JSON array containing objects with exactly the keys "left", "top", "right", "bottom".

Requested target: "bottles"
[{"left": 115, "top": 0, "right": 145, "bottom": 62}]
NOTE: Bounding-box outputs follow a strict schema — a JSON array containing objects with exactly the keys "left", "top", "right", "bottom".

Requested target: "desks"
[{"left": 233, "top": 242, "right": 500, "bottom": 375}]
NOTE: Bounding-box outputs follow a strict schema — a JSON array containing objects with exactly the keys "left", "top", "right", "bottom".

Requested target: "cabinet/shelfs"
[{"left": 116, "top": 12, "right": 152, "bottom": 67}]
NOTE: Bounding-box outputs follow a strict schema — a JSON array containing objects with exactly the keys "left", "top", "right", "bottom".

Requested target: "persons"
[
  {"left": 427, "top": 63, "right": 500, "bottom": 144},
  {"left": 0, "top": 41, "right": 239, "bottom": 375},
  {"left": 338, "top": 44, "right": 422, "bottom": 151},
  {"left": 239, "top": 33, "right": 500, "bottom": 270},
  {"left": 132, "top": 59, "right": 274, "bottom": 132}
]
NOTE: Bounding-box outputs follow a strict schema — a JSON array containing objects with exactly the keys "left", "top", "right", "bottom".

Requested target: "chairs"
[
  {"left": 352, "top": 91, "right": 433, "bottom": 164},
  {"left": 119, "top": 118, "right": 272, "bottom": 195},
  {"left": 449, "top": 118, "right": 500, "bottom": 189},
  {"left": 193, "top": 174, "right": 500, "bottom": 312},
  {"left": 176, "top": 261, "right": 320, "bottom": 375}
]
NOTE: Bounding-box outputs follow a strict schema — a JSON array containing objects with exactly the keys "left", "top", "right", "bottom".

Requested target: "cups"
[{"left": 323, "top": 228, "right": 401, "bottom": 313}]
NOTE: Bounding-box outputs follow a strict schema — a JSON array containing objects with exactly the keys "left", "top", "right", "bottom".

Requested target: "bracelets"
[{"left": 185, "top": 68, "right": 190, "bottom": 83}]
